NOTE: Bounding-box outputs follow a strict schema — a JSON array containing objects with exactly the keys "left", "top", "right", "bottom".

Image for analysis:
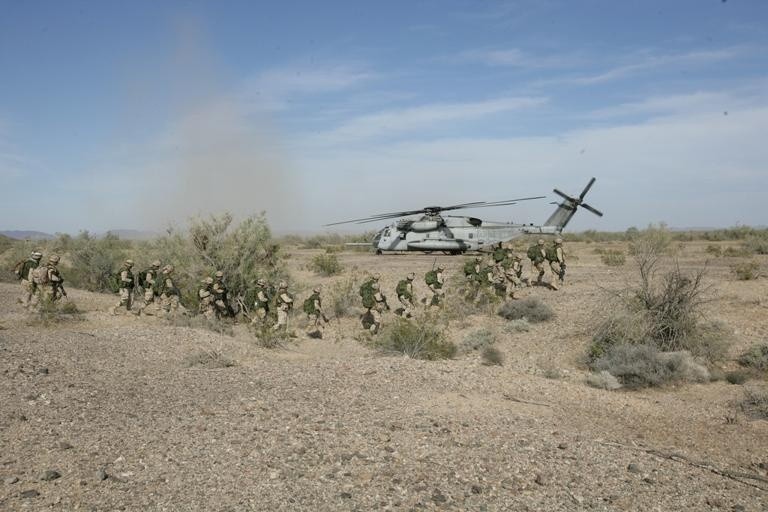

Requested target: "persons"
[
  {"left": 398, "top": 273, "right": 417, "bottom": 319},
  {"left": 302, "top": 288, "right": 326, "bottom": 340},
  {"left": 459, "top": 244, "right": 523, "bottom": 309},
  {"left": 109, "top": 259, "right": 137, "bottom": 316},
  {"left": 427, "top": 263, "right": 447, "bottom": 306},
  {"left": 212, "top": 271, "right": 230, "bottom": 316},
  {"left": 40, "top": 254, "right": 64, "bottom": 301},
  {"left": 526, "top": 239, "right": 546, "bottom": 287},
  {"left": 138, "top": 259, "right": 161, "bottom": 316},
  {"left": 359, "top": 273, "right": 384, "bottom": 334},
  {"left": 253, "top": 279, "right": 269, "bottom": 321},
  {"left": 21, "top": 252, "right": 42, "bottom": 303},
  {"left": 159, "top": 264, "right": 181, "bottom": 321},
  {"left": 550, "top": 238, "right": 566, "bottom": 290},
  {"left": 269, "top": 280, "right": 294, "bottom": 333},
  {"left": 199, "top": 276, "right": 214, "bottom": 319}
]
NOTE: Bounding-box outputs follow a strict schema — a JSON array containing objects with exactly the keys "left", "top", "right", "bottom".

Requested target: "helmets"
[
  {"left": 475, "top": 244, "right": 523, "bottom": 266},
  {"left": 372, "top": 264, "right": 447, "bottom": 281},
  {"left": 201, "top": 270, "right": 223, "bottom": 284},
  {"left": 30, "top": 250, "right": 60, "bottom": 264},
  {"left": 537, "top": 239, "right": 563, "bottom": 245},
  {"left": 257, "top": 278, "right": 321, "bottom": 293}
]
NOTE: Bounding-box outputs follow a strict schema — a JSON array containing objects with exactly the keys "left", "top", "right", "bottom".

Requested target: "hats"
[{"left": 122, "top": 257, "right": 175, "bottom": 272}]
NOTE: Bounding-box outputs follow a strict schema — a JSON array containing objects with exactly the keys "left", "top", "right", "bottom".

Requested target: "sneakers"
[
  {"left": 505, "top": 279, "right": 561, "bottom": 302},
  {"left": 108, "top": 308, "right": 164, "bottom": 317}
]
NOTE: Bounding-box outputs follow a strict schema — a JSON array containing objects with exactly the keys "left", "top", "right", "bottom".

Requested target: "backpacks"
[
  {"left": 464, "top": 262, "right": 490, "bottom": 282},
  {"left": 116, "top": 268, "right": 167, "bottom": 295},
  {"left": 14, "top": 260, "right": 51, "bottom": 284},
  {"left": 360, "top": 271, "right": 435, "bottom": 309},
  {"left": 243, "top": 287, "right": 319, "bottom": 314},
  {"left": 197, "top": 281, "right": 220, "bottom": 303},
  {"left": 527, "top": 246, "right": 558, "bottom": 262}
]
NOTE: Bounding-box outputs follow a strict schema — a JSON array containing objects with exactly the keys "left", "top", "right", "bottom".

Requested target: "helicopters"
[{"left": 322, "top": 173, "right": 607, "bottom": 258}]
